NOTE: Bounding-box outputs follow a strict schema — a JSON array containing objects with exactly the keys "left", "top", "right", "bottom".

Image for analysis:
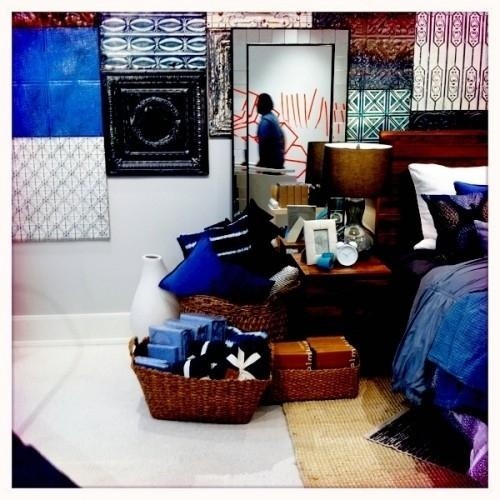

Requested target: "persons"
[{"left": 256, "top": 93, "right": 285, "bottom": 175}]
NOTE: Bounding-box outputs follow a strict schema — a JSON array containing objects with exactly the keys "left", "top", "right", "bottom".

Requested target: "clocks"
[{"left": 336, "top": 241, "right": 358, "bottom": 267}]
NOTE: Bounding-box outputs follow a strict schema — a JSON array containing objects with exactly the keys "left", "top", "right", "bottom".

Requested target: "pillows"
[{"left": 407, "top": 163, "right": 487, "bottom": 267}]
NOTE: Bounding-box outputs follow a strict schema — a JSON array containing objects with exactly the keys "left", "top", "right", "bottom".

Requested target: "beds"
[{"left": 378, "top": 129, "right": 488, "bottom": 486}]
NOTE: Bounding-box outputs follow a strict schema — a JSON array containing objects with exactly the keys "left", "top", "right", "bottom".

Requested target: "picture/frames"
[{"left": 303, "top": 219, "right": 337, "bottom": 266}]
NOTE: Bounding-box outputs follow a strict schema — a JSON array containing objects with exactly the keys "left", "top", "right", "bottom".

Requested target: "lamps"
[{"left": 322, "top": 142, "right": 393, "bottom": 262}]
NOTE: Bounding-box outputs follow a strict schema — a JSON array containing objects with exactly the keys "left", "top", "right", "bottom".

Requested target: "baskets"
[
  {"left": 178, "top": 294, "right": 286, "bottom": 344},
  {"left": 128, "top": 336, "right": 272, "bottom": 424},
  {"left": 261, "top": 351, "right": 360, "bottom": 402}
]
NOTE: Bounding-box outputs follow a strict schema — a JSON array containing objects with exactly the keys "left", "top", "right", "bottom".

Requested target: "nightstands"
[{"left": 287, "top": 249, "right": 391, "bottom": 380}]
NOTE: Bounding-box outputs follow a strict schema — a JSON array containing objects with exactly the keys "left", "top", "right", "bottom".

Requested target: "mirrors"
[{"left": 231, "top": 26, "right": 352, "bottom": 250}]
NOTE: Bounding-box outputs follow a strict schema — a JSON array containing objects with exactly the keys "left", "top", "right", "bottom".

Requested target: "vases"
[{"left": 129, "top": 254, "right": 179, "bottom": 343}]
